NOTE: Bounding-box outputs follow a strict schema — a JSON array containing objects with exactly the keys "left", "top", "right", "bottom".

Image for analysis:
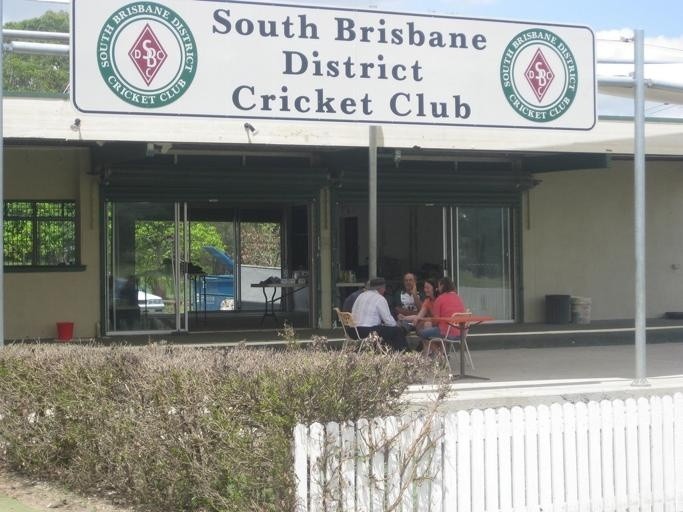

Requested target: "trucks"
[{"left": 192, "top": 245, "right": 235, "bottom": 311}]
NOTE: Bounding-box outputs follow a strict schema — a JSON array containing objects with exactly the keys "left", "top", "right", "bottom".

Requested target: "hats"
[{"left": 368, "top": 279, "right": 386, "bottom": 290}]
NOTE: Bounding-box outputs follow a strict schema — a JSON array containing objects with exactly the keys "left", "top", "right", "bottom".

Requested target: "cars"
[{"left": 137, "top": 290, "right": 165, "bottom": 311}]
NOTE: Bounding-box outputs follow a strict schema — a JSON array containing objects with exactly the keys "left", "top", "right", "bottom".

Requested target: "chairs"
[
  {"left": 428, "top": 313, "right": 474, "bottom": 372},
  {"left": 333, "top": 306, "right": 360, "bottom": 355},
  {"left": 340, "top": 311, "right": 387, "bottom": 355}
]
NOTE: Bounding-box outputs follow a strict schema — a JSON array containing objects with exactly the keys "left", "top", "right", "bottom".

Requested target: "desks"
[
  {"left": 431, "top": 316, "right": 489, "bottom": 381},
  {"left": 250, "top": 282, "right": 365, "bottom": 328}
]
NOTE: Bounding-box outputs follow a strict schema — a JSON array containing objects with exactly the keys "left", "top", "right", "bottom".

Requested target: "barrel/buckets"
[
  {"left": 56, "top": 322, "right": 73, "bottom": 340},
  {"left": 570, "top": 298, "right": 591, "bottom": 323}
]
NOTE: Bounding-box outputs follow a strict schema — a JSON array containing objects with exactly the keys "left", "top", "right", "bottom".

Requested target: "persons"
[
  {"left": 392, "top": 272, "right": 427, "bottom": 340},
  {"left": 411, "top": 277, "right": 440, "bottom": 352},
  {"left": 342, "top": 280, "right": 371, "bottom": 333},
  {"left": 348, "top": 278, "right": 411, "bottom": 353},
  {"left": 419, "top": 276, "right": 465, "bottom": 361}
]
